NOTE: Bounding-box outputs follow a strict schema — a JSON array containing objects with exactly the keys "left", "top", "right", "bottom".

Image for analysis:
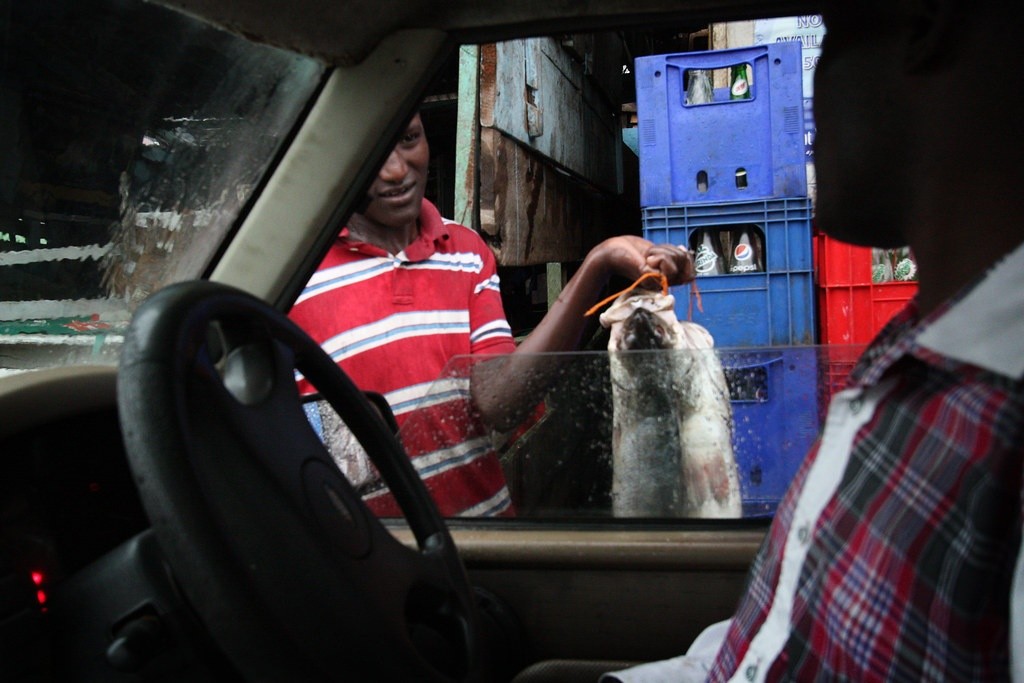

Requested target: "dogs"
[{"left": 604, "top": 303, "right": 743, "bottom": 521}]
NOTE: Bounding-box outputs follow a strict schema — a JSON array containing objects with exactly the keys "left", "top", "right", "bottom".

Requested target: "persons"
[
  {"left": 599, "top": 0, "right": 1022, "bottom": 681},
  {"left": 274, "top": 89, "right": 696, "bottom": 529}
]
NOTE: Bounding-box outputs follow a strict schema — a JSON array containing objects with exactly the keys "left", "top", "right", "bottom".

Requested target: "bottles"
[
  {"left": 892, "top": 245, "right": 921, "bottom": 282},
  {"left": 727, "top": 63, "right": 750, "bottom": 100},
  {"left": 870, "top": 247, "right": 894, "bottom": 283},
  {"left": 684, "top": 69, "right": 714, "bottom": 106},
  {"left": 725, "top": 225, "right": 762, "bottom": 274},
  {"left": 693, "top": 227, "right": 726, "bottom": 275}
]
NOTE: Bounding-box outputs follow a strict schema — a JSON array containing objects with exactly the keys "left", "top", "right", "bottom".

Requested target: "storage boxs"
[{"left": 632, "top": 16, "right": 925, "bottom": 517}]
